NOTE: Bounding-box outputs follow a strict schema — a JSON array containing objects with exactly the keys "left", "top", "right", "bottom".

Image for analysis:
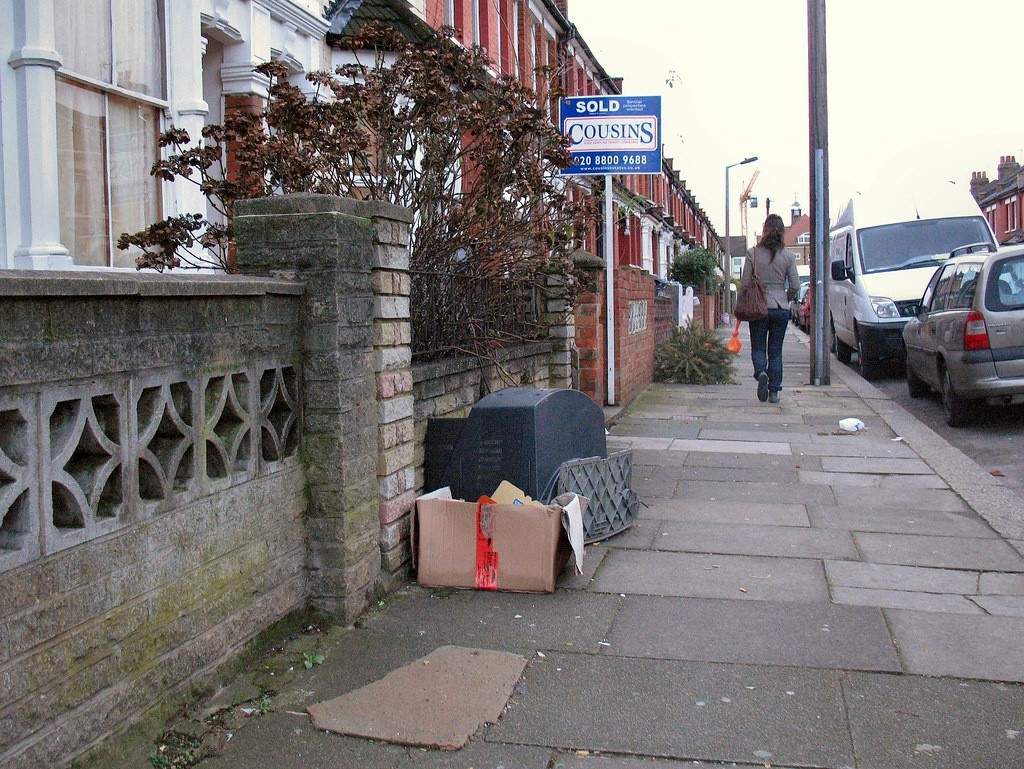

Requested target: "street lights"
[{"left": 723, "top": 155, "right": 759, "bottom": 314}]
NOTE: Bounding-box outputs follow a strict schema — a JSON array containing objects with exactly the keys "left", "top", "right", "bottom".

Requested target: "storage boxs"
[{"left": 411, "top": 486, "right": 590, "bottom": 594}]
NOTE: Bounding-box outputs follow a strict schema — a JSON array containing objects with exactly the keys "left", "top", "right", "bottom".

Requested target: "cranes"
[{"left": 738, "top": 170, "right": 759, "bottom": 250}]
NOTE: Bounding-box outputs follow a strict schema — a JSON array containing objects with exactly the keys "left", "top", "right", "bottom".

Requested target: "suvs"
[{"left": 900, "top": 241, "right": 1024, "bottom": 426}]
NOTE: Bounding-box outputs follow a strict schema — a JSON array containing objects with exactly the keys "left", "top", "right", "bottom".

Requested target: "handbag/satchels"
[
  {"left": 727, "top": 320, "right": 742, "bottom": 353},
  {"left": 734, "top": 247, "right": 769, "bottom": 322}
]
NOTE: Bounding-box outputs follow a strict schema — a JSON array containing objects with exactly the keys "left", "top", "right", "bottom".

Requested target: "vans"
[{"left": 829, "top": 175, "right": 1013, "bottom": 388}]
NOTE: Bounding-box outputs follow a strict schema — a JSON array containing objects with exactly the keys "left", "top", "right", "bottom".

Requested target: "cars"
[{"left": 787, "top": 279, "right": 810, "bottom": 335}]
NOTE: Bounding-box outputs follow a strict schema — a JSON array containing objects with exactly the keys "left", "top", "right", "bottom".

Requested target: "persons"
[{"left": 735, "top": 214, "right": 800, "bottom": 403}]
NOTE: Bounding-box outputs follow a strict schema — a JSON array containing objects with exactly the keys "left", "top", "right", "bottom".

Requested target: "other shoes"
[
  {"left": 768, "top": 392, "right": 781, "bottom": 403},
  {"left": 757, "top": 372, "right": 769, "bottom": 401}
]
